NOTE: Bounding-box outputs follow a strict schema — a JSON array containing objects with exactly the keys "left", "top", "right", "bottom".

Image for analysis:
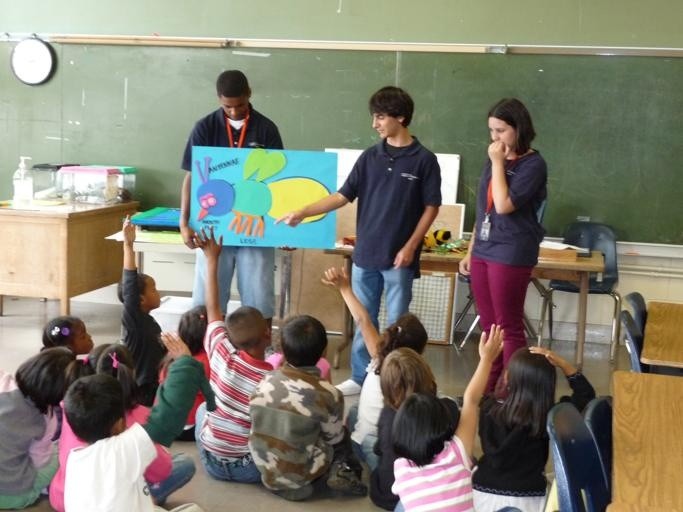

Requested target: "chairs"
[
  {"left": 620, "top": 309, "right": 651, "bottom": 373},
  {"left": 584, "top": 395, "right": 611, "bottom": 491},
  {"left": 546, "top": 401, "right": 610, "bottom": 512},
  {"left": 625, "top": 291, "right": 646, "bottom": 336},
  {"left": 454, "top": 201, "right": 547, "bottom": 349},
  {"left": 535, "top": 221, "right": 622, "bottom": 364}
]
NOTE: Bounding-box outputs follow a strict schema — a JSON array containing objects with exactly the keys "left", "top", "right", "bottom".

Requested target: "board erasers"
[{"left": 626, "top": 251, "right": 639, "bottom": 256}]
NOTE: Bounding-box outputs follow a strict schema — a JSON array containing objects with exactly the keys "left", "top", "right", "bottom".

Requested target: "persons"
[
  {"left": 459, "top": 99, "right": 547, "bottom": 406},
  {"left": 179, "top": 69, "right": 283, "bottom": 358},
  {"left": 193, "top": 225, "right": 276, "bottom": 484},
  {"left": 275, "top": 86, "right": 442, "bottom": 394},
  {"left": 265, "top": 315, "right": 333, "bottom": 384},
  {"left": 457, "top": 346, "right": 595, "bottom": 512},
  {"left": 248, "top": 315, "right": 368, "bottom": 502},
  {"left": 118, "top": 215, "right": 168, "bottom": 408},
  {"left": 3, "top": 346, "right": 77, "bottom": 509},
  {"left": 391, "top": 324, "right": 505, "bottom": 511},
  {"left": 370, "top": 347, "right": 437, "bottom": 510},
  {"left": 42, "top": 315, "right": 93, "bottom": 356},
  {"left": 321, "top": 266, "right": 428, "bottom": 472},
  {"left": 64, "top": 375, "right": 201, "bottom": 510},
  {"left": 49, "top": 330, "right": 196, "bottom": 510},
  {"left": 158, "top": 305, "right": 216, "bottom": 442}
]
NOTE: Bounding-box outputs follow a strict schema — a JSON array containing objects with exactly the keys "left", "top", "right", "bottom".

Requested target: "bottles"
[{"left": 424, "top": 230, "right": 454, "bottom": 249}]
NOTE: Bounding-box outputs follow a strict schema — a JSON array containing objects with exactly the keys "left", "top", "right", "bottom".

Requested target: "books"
[{"left": 335, "top": 379, "right": 362, "bottom": 426}]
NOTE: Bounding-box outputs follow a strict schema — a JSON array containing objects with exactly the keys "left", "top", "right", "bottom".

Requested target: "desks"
[
  {"left": 1, "top": 198, "right": 142, "bottom": 316},
  {"left": 639, "top": 300, "right": 683, "bottom": 369},
  {"left": 324, "top": 239, "right": 605, "bottom": 372},
  {"left": 101, "top": 227, "right": 297, "bottom": 370},
  {"left": 606, "top": 369, "right": 683, "bottom": 512}
]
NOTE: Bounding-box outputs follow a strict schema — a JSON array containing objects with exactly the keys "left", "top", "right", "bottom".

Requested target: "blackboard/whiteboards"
[{"left": 0, "top": 34, "right": 683, "bottom": 259}]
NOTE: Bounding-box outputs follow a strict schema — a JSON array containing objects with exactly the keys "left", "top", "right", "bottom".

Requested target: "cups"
[{"left": 56, "top": 172, "right": 78, "bottom": 204}]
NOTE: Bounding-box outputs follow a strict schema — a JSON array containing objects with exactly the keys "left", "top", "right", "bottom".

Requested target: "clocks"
[{"left": 10, "top": 37, "right": 58, "bottom": 85}]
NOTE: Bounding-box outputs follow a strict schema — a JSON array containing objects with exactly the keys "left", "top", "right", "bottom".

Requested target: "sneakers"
[
  {"left": 335, "top": 379, "right": 361, "bottom": 397},
  {"left": 327, "top": 463, "right": 368, "bottom": 498}
]
